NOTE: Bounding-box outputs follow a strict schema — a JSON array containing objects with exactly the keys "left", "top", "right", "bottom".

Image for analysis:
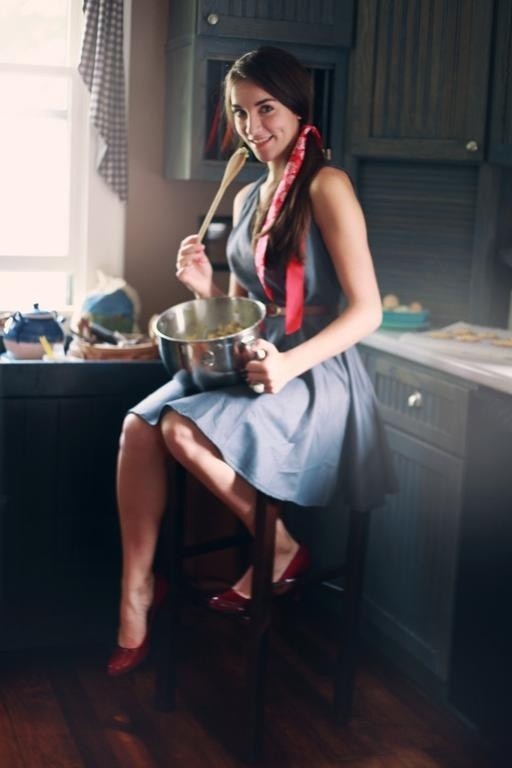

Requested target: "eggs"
[{"left": 382, "top": 294, "right": 421, "bottom": 312}]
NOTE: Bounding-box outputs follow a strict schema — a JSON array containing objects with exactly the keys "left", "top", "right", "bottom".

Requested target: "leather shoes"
[
  {"left": 207, "top": 544, "right": 311, "bottom": 615},
  {"left": 106, "top": 571, "right": 170, "bottom": 677}
]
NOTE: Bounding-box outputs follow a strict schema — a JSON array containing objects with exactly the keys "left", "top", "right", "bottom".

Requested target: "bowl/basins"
[{"left": 76, "top": 333, "right": 158, "bottom": 362}]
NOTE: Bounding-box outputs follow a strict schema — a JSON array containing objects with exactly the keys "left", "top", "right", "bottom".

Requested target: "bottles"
[{"left": 80, "top": 316, "right": 121, "bottom": 343}]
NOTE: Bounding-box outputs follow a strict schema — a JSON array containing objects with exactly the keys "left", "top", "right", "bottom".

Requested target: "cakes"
[{"left": 429, "top": 326, "right": 511, "bottom": 348}]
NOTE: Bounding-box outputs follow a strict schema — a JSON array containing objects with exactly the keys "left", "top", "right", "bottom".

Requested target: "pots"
[
  {"left": 1, "top": 305, "right": 64, "bottom": 358},
  {"left": 152, "top": 295, "right": 269, "bottom": 394}
]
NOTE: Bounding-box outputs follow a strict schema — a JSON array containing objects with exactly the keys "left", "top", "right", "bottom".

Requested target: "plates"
[{"left": 382, "top": 308, "right": 428, "bottom": 323}]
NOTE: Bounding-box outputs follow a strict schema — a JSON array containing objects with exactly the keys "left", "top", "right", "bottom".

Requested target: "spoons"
[{"left": 189, "top": 148, "right": 247, "bottom": 264}]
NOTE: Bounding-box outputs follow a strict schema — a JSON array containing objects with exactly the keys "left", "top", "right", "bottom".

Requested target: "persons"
[{"left": 97, "top": 40, "right": 386, "bottom": 677}]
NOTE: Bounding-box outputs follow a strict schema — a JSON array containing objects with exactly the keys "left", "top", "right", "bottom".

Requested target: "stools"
[{"left": 158, "top": 456, "right": 370, "bottom": 762}]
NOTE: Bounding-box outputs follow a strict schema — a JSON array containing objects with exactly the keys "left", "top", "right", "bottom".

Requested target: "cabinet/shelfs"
[
  {"left": 347, "top": 154, "right": 510, "bottom": 329},
  {"left": 162, "top": 36, "right": 353, "bottom": 182},
  {"left": 293, "top": 345, "right": 479, "bottom": 700},
  {"left": 164, "top": 3, "right": 359, "bottom": 51},
  {"left": 0, "top": 364, "right": 189, "bottom": 662},
  {"left": 350, "top": 1, "right": 509, "bottom": 164}
]
